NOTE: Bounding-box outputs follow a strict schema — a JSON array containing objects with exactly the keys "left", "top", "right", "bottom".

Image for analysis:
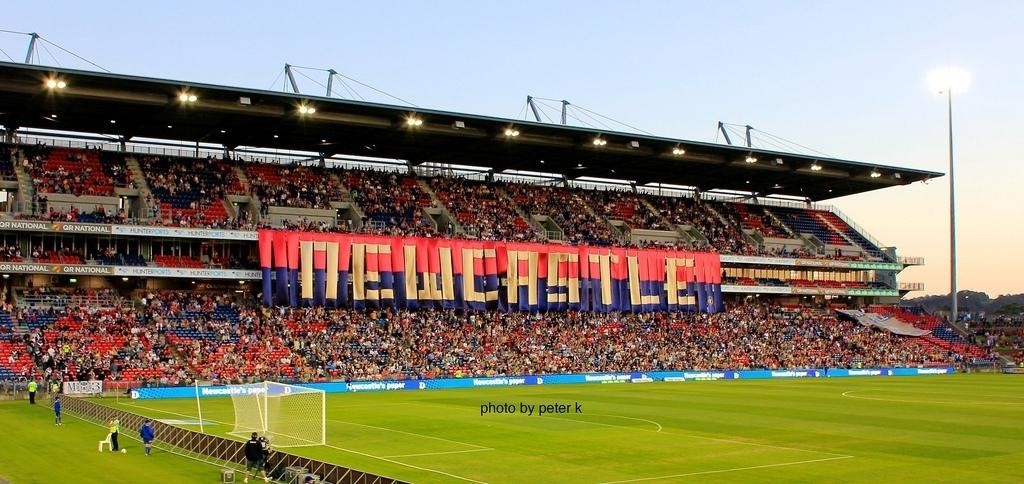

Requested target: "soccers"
[{"left": 120, "top": 448, "right": 127, "bottom": 453}]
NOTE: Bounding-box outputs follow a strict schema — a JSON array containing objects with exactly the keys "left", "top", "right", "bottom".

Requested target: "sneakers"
[
  {"left": 243, "top": 478, "right": 248, "bottom": 484},
  {"left": 264, "top": 476, "right": 274, "bottom": 484}
]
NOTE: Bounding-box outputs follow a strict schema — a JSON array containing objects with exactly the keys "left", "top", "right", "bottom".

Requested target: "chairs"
[{"left": 1, "top": 143, "right": 1024, "bottom": 390}]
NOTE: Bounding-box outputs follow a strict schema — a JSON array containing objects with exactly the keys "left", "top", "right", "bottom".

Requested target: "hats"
[
  {"left": 251, "top": 432, "right": 257, "bottom": 440},
  {"left": 305, "top": 477, "right": 315, "bottom": 481}
]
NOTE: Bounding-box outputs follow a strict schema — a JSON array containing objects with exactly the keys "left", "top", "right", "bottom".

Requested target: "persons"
[
  {"left": 2, "top": 135, "right": 1021, "bottom": 374},
  {"left": 26, "top": 377, "right": 37, "bottom": 404},
  {"left": 54, "top": 396, "right": 61, "bottom": 425},
  {"left": 344, "top": 375, "right": 354, "bottom": 392},
  {"left": 106, "top": 415, "right": 118, "bottom": 450},
  {"left": 138, "top": 418, "right": 154, "bottom": 456},
  {"left": 242, "top": 431, "right": 273, "bottom": 483}
]
